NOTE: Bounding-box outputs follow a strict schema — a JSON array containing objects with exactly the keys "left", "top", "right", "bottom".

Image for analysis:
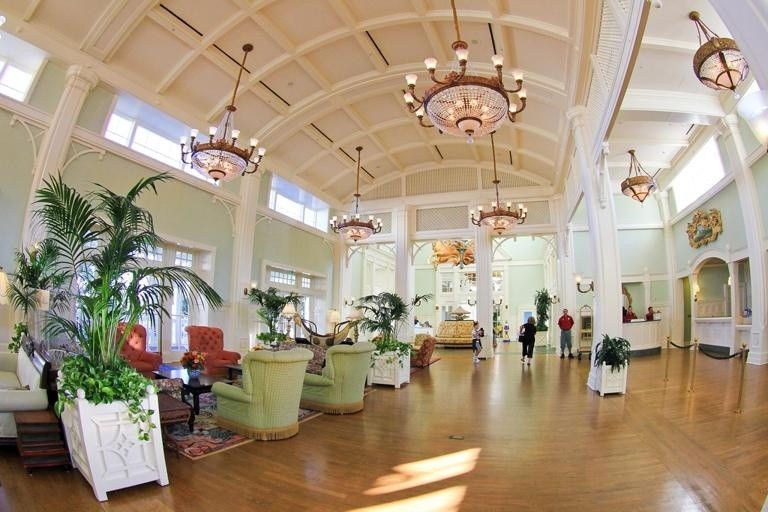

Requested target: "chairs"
[
  {"left": 299, "top": 341, "right": 377, "bottom": 414},
  {"left": 211, "top": 347, "right": 314, "bottom": 441},
  {"left": 410, "top": 333, "right": 429, "bottom": 350},
  {"left": 114, "top": 323, "right": 162, "bottom": 373},
  {"left": 410, "top": 337, "right": 436, "bottom": 368},
  {"left": 185, "top": 325, "right": 241, "bottom": 376},
  {"left": 152, "top": 379, "right": 195, "bottom": 433}
]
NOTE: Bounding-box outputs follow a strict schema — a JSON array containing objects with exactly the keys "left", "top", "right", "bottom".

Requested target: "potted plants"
[
  {"left": 29, "top": 171, "right": 223, "bottom": 503},
  {"left": 534, "top": 289, "right": 552, "bottom": 347},
  {"left": 594, "top": 334, "right": 631, "bottom": 396},
  {"left": 352, "top": 291, "right": 434, "bottom": 388}
]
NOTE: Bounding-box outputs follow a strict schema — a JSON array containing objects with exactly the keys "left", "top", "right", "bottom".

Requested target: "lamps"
[
  {"left": 330, "top": 146, "right": 383, "bottom": 242},
  {"left": 576, "top": 276, "right": 594, "bottom": 293},
  {"left": 621, "top": 150, "right": 657, "bottom": 203},
  {"left": 36, "top": 289, "right": 50, "bottom": 311},
  {"left": 468, "top": 131, "right": 528, "bottom": 235},
  {"left": 179, "top": 44, "right": 266, "bottom": 182},
  {"left": 0, "top": 266, "right": 10, "bottom": 305},
  {"left": 283, "top": 303, "right": 296, "bottom": 339},
  {"left": 493, "top": 296, "right": 503, "bottom": 305},
  {"left": 689, "top": 10, "right": 749, "bottom": 93},
  {"left": 344, "top": 297, "right": 356, "bottom": 307},
  {"left": 550, "top": 294, "right": 560, "bottom": 304},
  {"left": 468, "top": 299, "right": 477, "bottom": 306},
  {"left": 330, "top": 309, "right": 339, "bottom": 334},
  {"left": 404, "top": 1, "right": 526, "bottom": 138}
]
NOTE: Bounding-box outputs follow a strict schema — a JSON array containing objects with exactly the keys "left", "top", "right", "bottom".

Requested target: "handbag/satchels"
[{"left": 519, "top": 336, "right": 526, "bottom": 342}]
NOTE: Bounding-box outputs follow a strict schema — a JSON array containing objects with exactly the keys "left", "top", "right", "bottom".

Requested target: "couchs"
[
  {"left": 433, "top": 321, "right": 474, "bottom": 348},
  {"left": 280, "top": 342, "right": 326, "bottom": 374},
  {"left": 0, "top": 335, "right": 52, "bottom": 438}
]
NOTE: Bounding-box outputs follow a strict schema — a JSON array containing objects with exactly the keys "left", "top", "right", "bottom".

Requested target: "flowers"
[{"left": 180, "top": 350, "right": 205, "bottom": 368}]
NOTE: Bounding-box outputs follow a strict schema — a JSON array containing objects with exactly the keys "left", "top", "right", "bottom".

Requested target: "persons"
[
  {"left": 624, "top": 306, "right": 637, "bottom": 321},
  {"left": 472, "top": 322, "right": 484, "bottom": 364},
  {"left": 646, "top": 307, "right": 661, "bottom": 321},
  {"left": 339, "top": 338, "right": 353, "bottom": 345},
  {"left": 558, "top": 309, "right": 574, "bottom": 359},
  {"left": 519, "top": 317, "right": 536, "bottom": 365}
]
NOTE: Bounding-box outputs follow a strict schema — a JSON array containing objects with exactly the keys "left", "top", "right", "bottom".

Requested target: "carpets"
[{"left": 167, "top": 356, "right": 442, "bottom": 460}]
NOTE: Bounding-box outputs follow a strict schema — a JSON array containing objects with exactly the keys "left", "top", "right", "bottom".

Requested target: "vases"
[{"left": 187, "top": 366, "right": 200, "bottom": 378}]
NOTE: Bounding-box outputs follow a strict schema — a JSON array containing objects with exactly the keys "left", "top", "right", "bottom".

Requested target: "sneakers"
[
  {"left": 473, "top": 358, "right": 481, "bottom": 364},
  {"left": 521, "top": 359, "right": 531, "bottom": 365},
  {"left": 560, "top": 353, "right": 574, "bottom": 359}
]
